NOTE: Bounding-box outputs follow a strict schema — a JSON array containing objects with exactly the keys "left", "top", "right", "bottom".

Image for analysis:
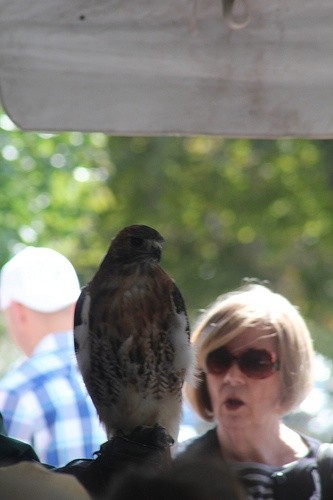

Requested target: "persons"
[
  {"left": 1, "top": 246, "right": 102, "bottom": 464},
  {"left": 173, "top": 282, "right": 333, "bottom": 500}
]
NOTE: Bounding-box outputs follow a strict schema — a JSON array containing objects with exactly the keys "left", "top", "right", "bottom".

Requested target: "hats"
[{"left": 0, "top": 246, "right": 83, "bottom": 313}]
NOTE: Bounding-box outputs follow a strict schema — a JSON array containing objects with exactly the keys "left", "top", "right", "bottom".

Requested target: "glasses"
[{"left": 200, "top": 347, "right": 285, "bottom": 381}]
